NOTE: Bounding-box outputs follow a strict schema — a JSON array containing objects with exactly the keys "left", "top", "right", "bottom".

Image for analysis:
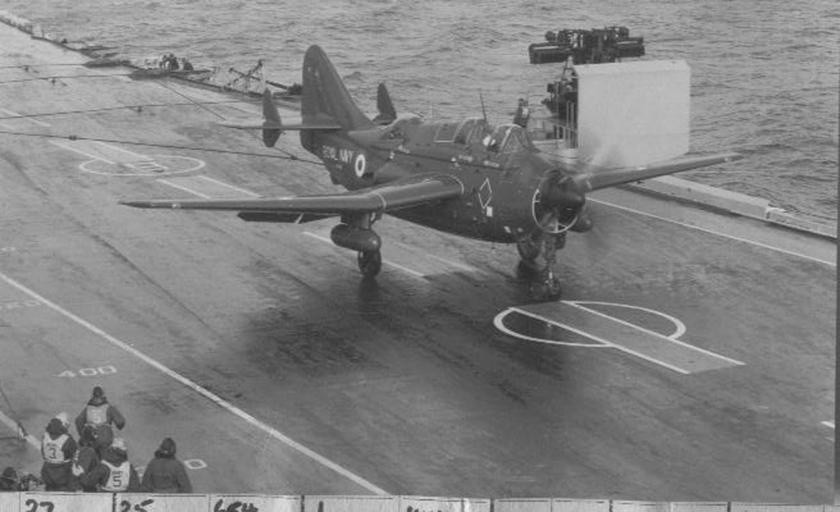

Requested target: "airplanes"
[{"left": 116, "top": 40, "right": 754, "bottom": 300}]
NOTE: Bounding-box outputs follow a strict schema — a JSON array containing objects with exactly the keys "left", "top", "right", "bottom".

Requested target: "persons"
[
  {"left": 158, "top": 51, "right": 194, "bottom": 73},
  {"left": 512, "top": 94, "right": 531, "bottom": 126},
  {"left": 1, "top": 411, "right": 193, "bottom": 493},
  {"left": 75, "top": 385, "right": 125, "bottom": 435}
]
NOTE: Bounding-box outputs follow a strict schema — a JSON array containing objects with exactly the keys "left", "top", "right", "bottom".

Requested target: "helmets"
[
  {"left": 94, "top": 387, "right": 102, "bottom": 395},
  {"left": 81, "top": 426, "right": 98, "bottom": 443},
  {"left": 111, "top": 437, "right": 125, "bottom": 449},
  {"left": 54, "top": 413, "right": 71, "bottom": 428},
  {"left": 158, "top": 438, "right": 175, "bottom": 454}
]
